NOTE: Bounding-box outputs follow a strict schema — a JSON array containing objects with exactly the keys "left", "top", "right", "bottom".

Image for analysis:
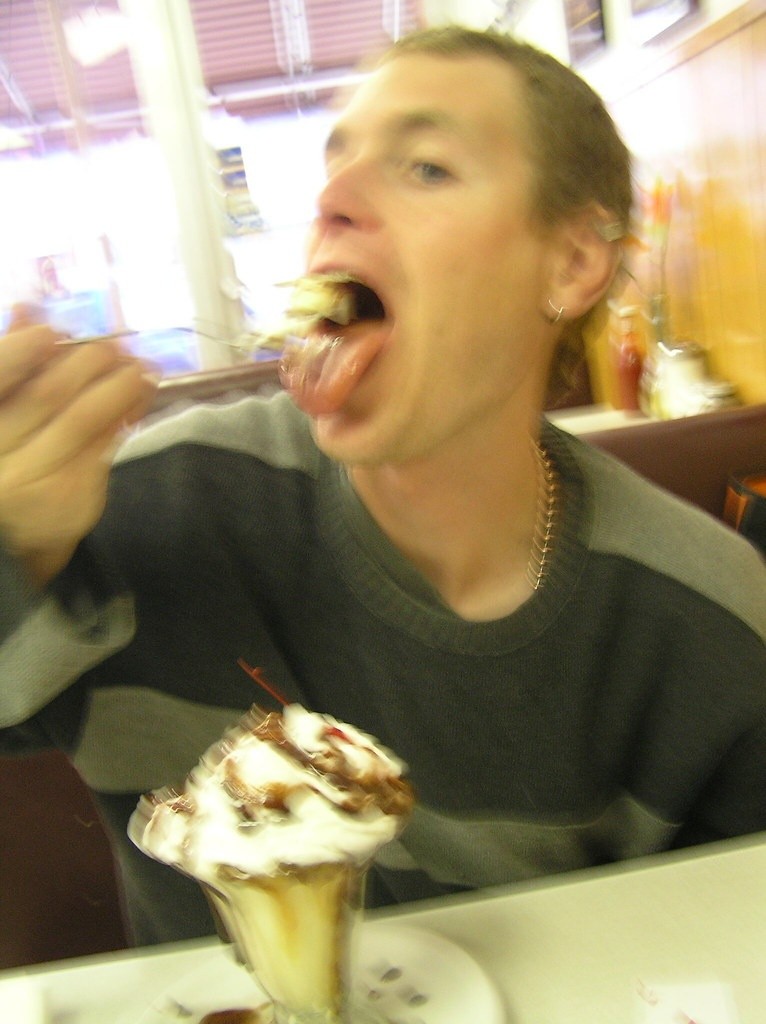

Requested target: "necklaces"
[{"left": 529, "top": 442, "right": 561, "bottom": 588}]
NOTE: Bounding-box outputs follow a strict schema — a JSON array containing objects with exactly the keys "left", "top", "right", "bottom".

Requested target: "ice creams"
[
  {"left": 129, "top": 700, "right": 413, "bottom": 1024},
  {"left": 286, "top": 278, "right": 356, "bottom": 323}
]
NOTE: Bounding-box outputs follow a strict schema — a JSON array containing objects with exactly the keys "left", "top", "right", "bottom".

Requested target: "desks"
[
  {"left": 2, "top": 833, "right": 766, "bottom": 1024},
  {"left": 553, "top": 407, "right": 660, "bottom": 436}
]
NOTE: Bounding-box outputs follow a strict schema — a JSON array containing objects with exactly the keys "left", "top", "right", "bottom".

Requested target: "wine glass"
[{"left": 125, "top": 778, "right": 416, "bottom": 1024}]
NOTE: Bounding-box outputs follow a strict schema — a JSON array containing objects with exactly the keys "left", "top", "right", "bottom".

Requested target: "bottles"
[{"left": 617, "top": 305, "right": 641, "bottom": 413}]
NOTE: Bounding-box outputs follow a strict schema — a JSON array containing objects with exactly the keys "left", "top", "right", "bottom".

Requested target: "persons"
[{"left": 1, "top": 23, "right": 766, "bottom": 942}]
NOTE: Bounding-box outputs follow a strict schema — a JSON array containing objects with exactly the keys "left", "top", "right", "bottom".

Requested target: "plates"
[{"left": 139, "top": 920, "right": 507, "bottom": 1024}]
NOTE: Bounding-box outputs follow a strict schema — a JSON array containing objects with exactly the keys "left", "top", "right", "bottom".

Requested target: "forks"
[{"left": 57, "top": 311, "right": 325, "bottom": 347}]
